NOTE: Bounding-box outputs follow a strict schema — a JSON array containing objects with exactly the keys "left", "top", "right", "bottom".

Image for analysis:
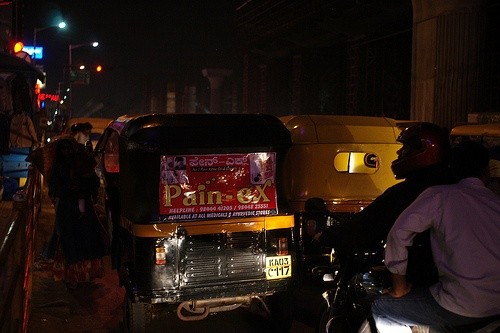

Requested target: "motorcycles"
[
  {"left": 58, "top": 113, "right": 302, "bottom": 333},
  {"left": 303, "top": 196, "right": 500, "bottom": 333},
  {"left": 275, "top": 114, "right": 406, "bottom": 266}
]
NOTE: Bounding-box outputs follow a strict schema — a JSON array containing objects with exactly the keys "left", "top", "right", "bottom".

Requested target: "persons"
[
  {"left": 315, "top": 121, "right": 500, "bottom": 333},
  {"left": 40, "top": 122, "right": 97, "bottom": 268},
  {"left": 9, "top": 103, "right": 39, "bottom": 188}
]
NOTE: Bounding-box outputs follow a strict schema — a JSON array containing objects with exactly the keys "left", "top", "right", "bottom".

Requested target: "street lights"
[
  {"left": 30, "top": 21, "right": 68, "bottom": 119},
  {"left": 68, "top": 40, "right": 99, "bottom": 118}
]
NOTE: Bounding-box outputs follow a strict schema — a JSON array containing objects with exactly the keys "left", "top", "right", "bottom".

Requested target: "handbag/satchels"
[{"left": 25, "top": 139, "right": 58, "bottom": 175}]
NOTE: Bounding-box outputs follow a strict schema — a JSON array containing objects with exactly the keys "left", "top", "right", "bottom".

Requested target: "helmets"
[{"left": 391, "top": 122, "right": 449, "bottom": 179}]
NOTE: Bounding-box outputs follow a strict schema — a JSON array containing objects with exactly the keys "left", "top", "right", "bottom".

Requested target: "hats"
[{"left": 71, "top": 122, "right": 93, "bottom": 133}]
